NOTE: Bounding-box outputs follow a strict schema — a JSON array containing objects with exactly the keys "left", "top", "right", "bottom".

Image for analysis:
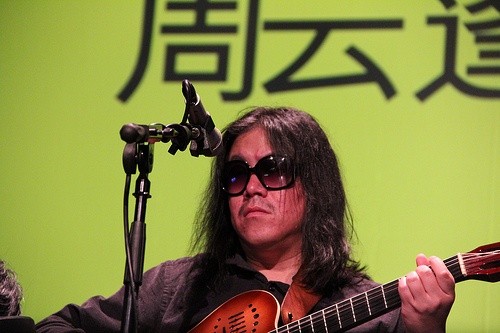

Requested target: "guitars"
[{"left": 186, "top": 240, "right": 500, "bottom": 333}]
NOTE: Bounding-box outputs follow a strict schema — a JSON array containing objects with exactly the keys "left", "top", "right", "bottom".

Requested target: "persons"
[
  {"left": 34, "top": 106, "right": 455, "bottom": 333},
  {"left": 0, "top": 259, "right": 24, "bottom": 318}
]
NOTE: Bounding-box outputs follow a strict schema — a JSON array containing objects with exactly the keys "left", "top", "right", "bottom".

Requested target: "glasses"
[{"left": 217, "top": 154, "right": 302, "bottom": 196}]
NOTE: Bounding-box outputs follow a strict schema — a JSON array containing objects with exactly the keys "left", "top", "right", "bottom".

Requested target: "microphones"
[{"left": 182, "top": 80, "right": 224, "bottom": 157}]
